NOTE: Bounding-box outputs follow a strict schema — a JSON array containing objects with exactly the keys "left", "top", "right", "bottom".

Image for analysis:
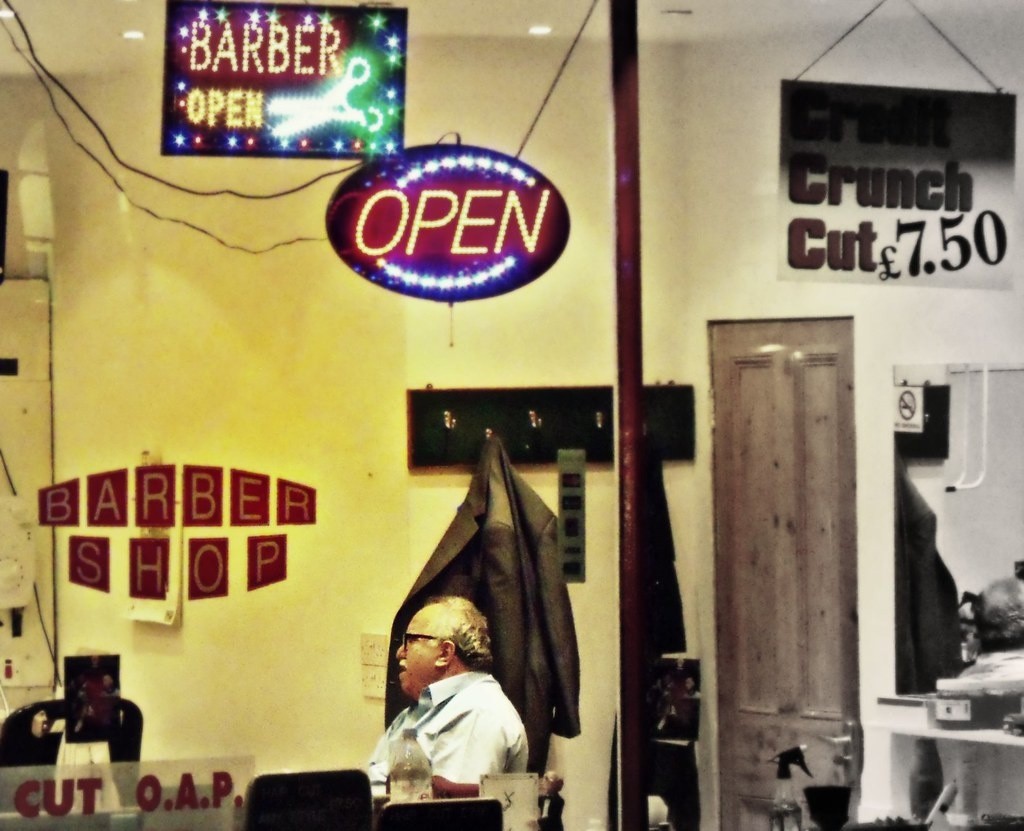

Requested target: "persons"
[
  {"left": 96, "top": 675, "right": 119, "bottom": 700},
  {"left": 362, "top": 599, "right": 529, "bottom": 801}
]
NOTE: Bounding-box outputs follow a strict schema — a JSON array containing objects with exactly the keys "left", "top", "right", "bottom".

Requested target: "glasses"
[{"left": 404, "top": 633, "right": 438, "bottom": 649}]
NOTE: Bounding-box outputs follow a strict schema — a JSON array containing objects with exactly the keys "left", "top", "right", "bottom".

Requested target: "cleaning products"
[
  {"left": 907, "top": 734, "right": 981, "bottom": 822},
  {"left": 769, "top": 744, "right": 815, "bottom": 830}
]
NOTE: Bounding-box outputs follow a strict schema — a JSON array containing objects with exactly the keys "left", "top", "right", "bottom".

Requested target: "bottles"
[{"left": 389, "top": 728, "right": 432, "bottom": 805}]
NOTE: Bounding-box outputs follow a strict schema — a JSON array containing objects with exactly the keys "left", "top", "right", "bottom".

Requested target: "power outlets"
[{"left": 359, "top": 634, "right": 389, "bottom": 699}]
